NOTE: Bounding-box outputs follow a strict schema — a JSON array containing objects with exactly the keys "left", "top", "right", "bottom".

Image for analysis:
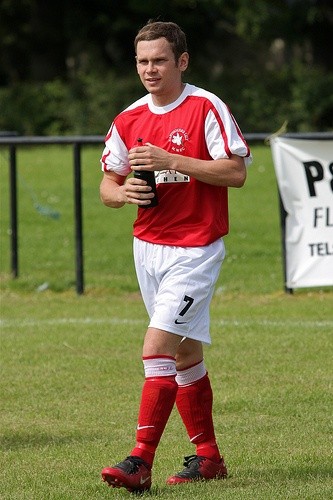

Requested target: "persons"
[{"left": 100, "top": 22, "right": 252, "bottom": 493}]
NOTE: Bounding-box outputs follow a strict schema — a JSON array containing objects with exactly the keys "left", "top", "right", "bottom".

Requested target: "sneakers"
[
  {"left": 101, "top": 455, "right": 152, "bottom": 493},
  {"left": 168, "top": 454, "right": 228, "bottom": 485}
]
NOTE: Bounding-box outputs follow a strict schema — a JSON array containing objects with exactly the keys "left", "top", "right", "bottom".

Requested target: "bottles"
[{"left": 134, "top": 137, "right": 159, "bottom": 208}]
